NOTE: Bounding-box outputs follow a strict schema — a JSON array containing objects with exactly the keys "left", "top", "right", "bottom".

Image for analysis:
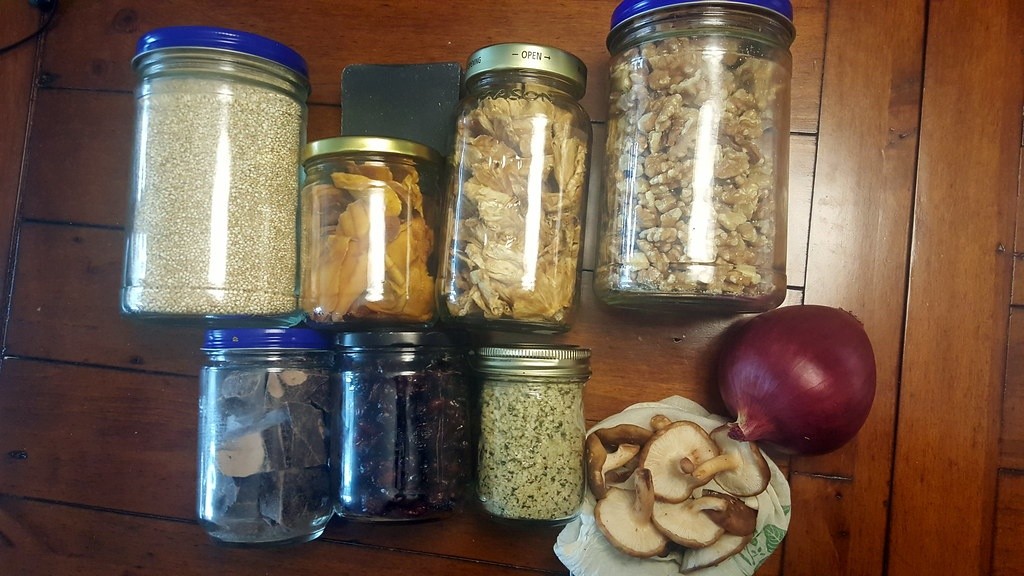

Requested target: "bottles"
[
  {"left": 195, "top": 325, "right": 336, "bottom": 547},
  {"left": 299, "top": 135, "right": 440, "bottom": 333},
  {"left": 332, "top": 328, "right": 476, "bottom": 527},
  {"left": 433, "top": 42, "right": 593, "bottom": 337},
  {"left": 471, "top": 343, "right": 593, "bottom": 527},
  {"left": 595, "top": 0, "right": 797, "bottom": 314},
  {"left": 120, "top": 25, "right": 312, "bottom": 330}
]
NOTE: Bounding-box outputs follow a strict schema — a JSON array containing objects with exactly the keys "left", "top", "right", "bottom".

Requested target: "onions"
[{"left": 717, "top": 303, "right": 877, "bottom": 458}]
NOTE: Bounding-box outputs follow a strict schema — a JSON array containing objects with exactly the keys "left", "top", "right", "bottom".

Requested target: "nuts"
[{"left": 596, "top": 19, "right": 788, "bottom": 298}]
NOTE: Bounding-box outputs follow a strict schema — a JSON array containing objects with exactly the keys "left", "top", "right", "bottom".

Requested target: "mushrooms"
[{"left": 583, "top": 415, "right": 772, "bottom": 572}]
